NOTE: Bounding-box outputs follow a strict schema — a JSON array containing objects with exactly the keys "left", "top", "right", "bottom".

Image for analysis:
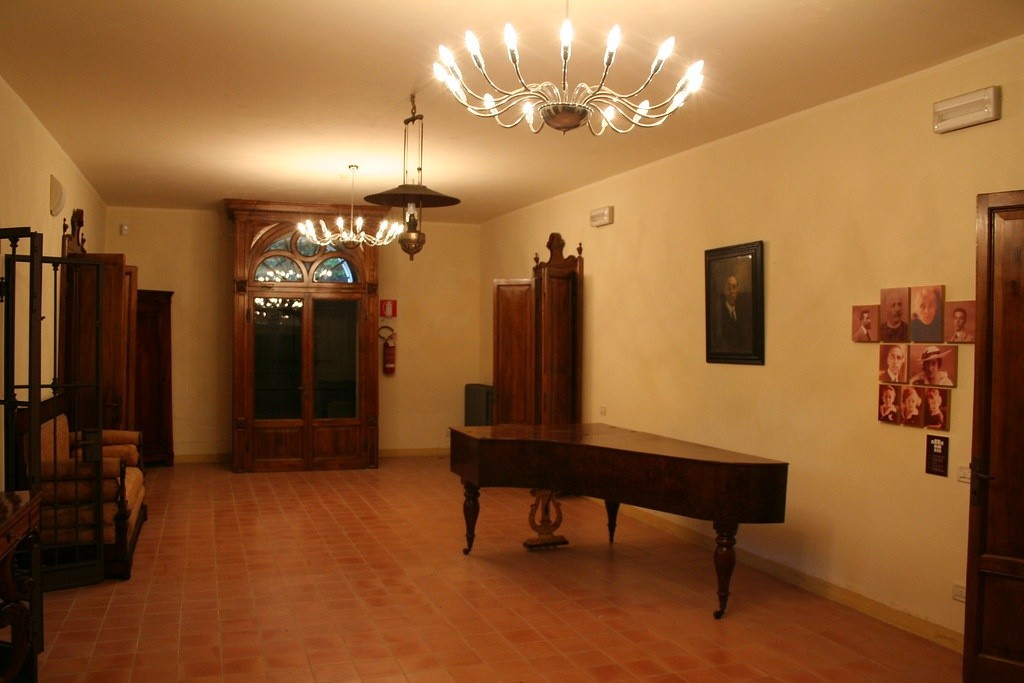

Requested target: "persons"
[
  {"left": 924, "top": 389, "right": 943, "bottom": 429},
  {"left": 720, "top": 275, "right": 744, "bottom": 341},
  {"left": 880, "top": 292, "right": 907, "bottom": 342},
  {"left": 901, "top": 387, "right": 921, "bottom": 424},
  {"left": 879, "top": 385, "right": 898, "bottom": 421},
  {"left": 910, "top": 345, "right": 953, "bottom": 386},
  {"left": 946, "top": 309, "right": 974, "bottom": 342},
  {"left": 910, "top": 287, "right": 943, "bottom": 342},
  {"left": 853, "top": 310, "right": 872, "bottom": 342},
  {"left": 880, "top": 346, "right": 905, "bottom": 382}
]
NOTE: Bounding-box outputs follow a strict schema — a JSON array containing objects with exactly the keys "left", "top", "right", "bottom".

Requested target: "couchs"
[{"left": 22, "top": 415, "right": 149, "bottom": 582}]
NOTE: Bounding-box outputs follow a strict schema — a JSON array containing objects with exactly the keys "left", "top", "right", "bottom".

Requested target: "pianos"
[{"left": 448, "top": 420, "right": 791, "bottom": 620}]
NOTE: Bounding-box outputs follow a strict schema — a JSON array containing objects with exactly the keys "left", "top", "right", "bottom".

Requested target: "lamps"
[
  {"left": 298, "top": 165, "right": 404, "bottom": 253},
  {"left": 50, "top": 175, "right": 66, "bottom": 216},
  {"left": 933, "top": 85, "right": 1001, "bottom": 132},
  {"left": 432, "top": 0, "right": 705, "bottom": 136},
  {"left": 590, "top": 206, "right": 614, "bottom": 227},
  {"left": 364, "top": 94, "right": 461, "bottom": 262}
]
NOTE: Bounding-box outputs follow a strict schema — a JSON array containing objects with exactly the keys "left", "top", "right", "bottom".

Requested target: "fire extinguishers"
[{"left": 378, "top": 326, "right": 397, "bottom": 375}]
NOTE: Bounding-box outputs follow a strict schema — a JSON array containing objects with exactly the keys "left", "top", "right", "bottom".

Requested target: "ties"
[
  {"left": 866, "top": 331, "right": 870, "bottom": 341},
  {"left": 953, "top": 334, "right": 958, "bottom": 342},
  {"left": 731, "top": 310, "right": 736, "bottom": 322}
]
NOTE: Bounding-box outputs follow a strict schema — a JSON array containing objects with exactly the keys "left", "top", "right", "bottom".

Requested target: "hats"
[
  {"left": 903, "top": 388, "right": 916, "bottom": 402},
  {"left": 917, "top": 346, "right": 953, "bottom": 362}
]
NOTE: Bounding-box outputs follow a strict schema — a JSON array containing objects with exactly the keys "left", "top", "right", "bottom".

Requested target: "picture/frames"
[{"left": 703, "top": 240, "right": 765, "bottom": 367}]
